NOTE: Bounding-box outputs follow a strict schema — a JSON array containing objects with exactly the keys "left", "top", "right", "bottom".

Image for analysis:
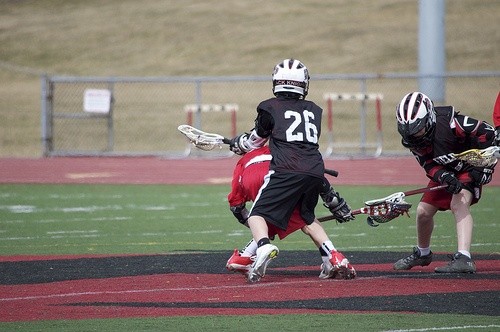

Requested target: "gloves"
[
  {"left": 229, "top": 132, "right": 249, "bottom": 156},
  {"left": 469, "top": 168, "right": 494, "bottom": 186},
  {"left": 440, "top": 175, "right": 462, "bottom": 194},
  {"left": 323, "top": 194, "right": 356, "bottom": 223}
]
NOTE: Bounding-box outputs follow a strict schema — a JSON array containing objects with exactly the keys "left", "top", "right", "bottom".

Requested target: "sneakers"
[
  {"left": 434, "top": 253, "right": 477, "bottom": 274},
  {"left": 394, "top": 246, "right": 433, "bottom": 270},
  {"left": 249, "top": 245, "right": 279, "bottom": 281},
  {"left": 329, "top": 250, "right": 357, "bottom": 280},
  {"left": 318, "top": 256, "right": 338, "bottom": 279},
  {"left": 225, "top": 249, "right": 254, "bottom": 278}
]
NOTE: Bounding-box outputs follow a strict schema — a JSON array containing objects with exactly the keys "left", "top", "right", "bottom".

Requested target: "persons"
[
  {"left": 226, "top": 59, "right": 358, "bottom": 280},
  {"left": 392, "top": 91, "right": 500, "bottom": 273}
]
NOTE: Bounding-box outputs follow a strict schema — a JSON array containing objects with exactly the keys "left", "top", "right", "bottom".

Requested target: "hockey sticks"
[
  {"left": 364, "top": 179, "right": 474, "bottom": 205},
  {"left": 178, "top": 125, "right": 338, "bottom": 177},
  {"left": 316, "top": 202, "right": 411, "bottom": 227}
]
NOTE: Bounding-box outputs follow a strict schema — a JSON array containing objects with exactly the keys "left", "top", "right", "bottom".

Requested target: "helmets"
[
  {"left": 272, "top": 59, "right": 309, "bottom": 99},
  {"left": 395, "top": 92, "right": 437, "bottom": 128}
]
